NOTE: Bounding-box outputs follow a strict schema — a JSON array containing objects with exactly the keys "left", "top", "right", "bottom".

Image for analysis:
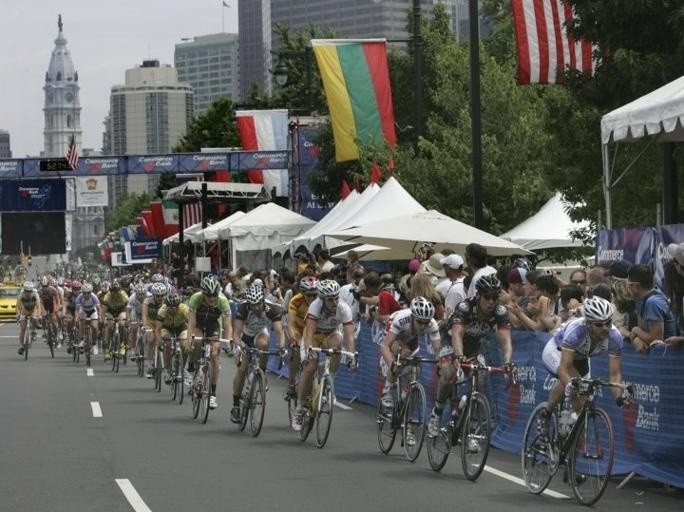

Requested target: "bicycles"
[
  {"left": 230, "top": 342, "right": 288, "bottom": 438},
  {"left": 520, "top": 374, "right": 640, "bottom": 507},
  {"left": 284, "top": 342, "right": 361, "bottom": 449},
  {"left": 425, "top": 353, "right": 519, "bottom": 484},
  {"left": 10, "top": 314, "right": 239, "bottom": 422},
  {"left": 374, "top": 346, "right": 441, "bottom": 463}
]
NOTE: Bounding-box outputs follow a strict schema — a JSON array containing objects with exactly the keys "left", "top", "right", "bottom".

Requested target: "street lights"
[{"left": 271, "top": 32, "right": 422, "bottom": 160}]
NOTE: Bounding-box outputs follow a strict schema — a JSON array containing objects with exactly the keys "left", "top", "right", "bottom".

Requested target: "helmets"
[
  {"left": 22, "top": 272, "right": 181, "bottom": 307},
  {"left": 476, "top": 276, "right": 502, "bottom": 296},
  {"left": 317, "top": 279, "right": 340, "bottom": 299},
  {"left": 409, "top": 296, "right": 435, "bottom": 322},
  {"left": 245, "top": 285, "right": 264, "bottom": 304},
  {"left": 299, "top": 274, "right": 320, "bottom": 294},
  {"left": 584, "top": 294, "right": 614, "bottom": 325},
  {"left": 200, "top": 276, "right": 220, "bottom": 295}
]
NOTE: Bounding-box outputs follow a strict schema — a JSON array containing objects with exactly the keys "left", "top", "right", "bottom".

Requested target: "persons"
[{"left": 16, "top": 244, "right": 684, "bottom": 484}]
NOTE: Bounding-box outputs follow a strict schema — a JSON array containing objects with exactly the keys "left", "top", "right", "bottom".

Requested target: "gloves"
[
  {"left": 452, "top": 354, "right": 468, "bottom": 369},
  {"left": 157, "top": 336, "right": 234, "bottom": 356},
  {"left": 565, "top": 383, "right": 578, "bottom": 397},
  {"left": 345, "top": 357, "right": 359, "bottom": 371},
  {"left": 387, "top": 361, "right": 402, "bottom": 376},
  {"left": 616, "top": 395, "right": 629, "bottom": 408},
  {"left": 504, "top": 360, "right": 520, "bottom": 376},
  {"left": 279, "top": 338, "right": 298, "bottom": 358}
]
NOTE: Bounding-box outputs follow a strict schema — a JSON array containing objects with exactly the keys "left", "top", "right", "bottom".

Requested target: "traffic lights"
[
  {"left": 27, "top": 256, "right": 32, "bottom": 267},
  {"left": 18, "top": 255, "right": 24, "bottom": 266}
]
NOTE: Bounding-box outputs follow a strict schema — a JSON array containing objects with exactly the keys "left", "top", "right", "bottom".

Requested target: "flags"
[
  {"left": 234, "top": 108, "right": 290, "bottom": 197},
  {"left": 511, "top": 0, "right": 609, "bottom": 86},
  {"left": 200, "top": 147, "right": 242, "bottom": 214},
  {"left": 65, "top": 133, "right": 80, "bottom": 169},
  {"left": 98, "top": 173, "right": 205, "bottom": 268},
  {"left": 309, "top": 38, "right": 397, "bottom": 163}
]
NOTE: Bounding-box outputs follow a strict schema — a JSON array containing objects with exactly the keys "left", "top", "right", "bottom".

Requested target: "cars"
[{"left": 0, "top": 285, "right": 25, "bottom": 325}]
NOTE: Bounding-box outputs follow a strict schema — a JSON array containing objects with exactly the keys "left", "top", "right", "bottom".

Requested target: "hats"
[
  {"left": 507, "top": 266, "right": 527, "bottom": 282},
  {"left": 410, "top": 252, "right": 464, "bottom": 275},
  {"left": 667, "top": 242, "right": 684, "bottom": 266}
]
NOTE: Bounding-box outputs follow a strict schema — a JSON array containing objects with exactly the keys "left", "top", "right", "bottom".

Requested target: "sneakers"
[
  {"left": 20, "top": 331, "right": 143, "bottom": 362},
  {"left": 402, "top": 421, "right": 415, "bottom": 446},
  {"left": 427, "top": 407, "right": 442, "bottom": 437},
  {"left": 230, "top": 404, "right": 241, "bottom": 424},
  {"left": 291, "top": 403, "right": 306, "bottom": 431},
  {"left": 468, "top": 438, "right": 482, "bottom": 453},
  {"left": 207, "top": 392, "right": 217, "bottom": 409},
  {"left": 380, "top": 385, "right": 394, "bottom": 407},
  {"left": 537, "top": 408, "right": 550, "bottom": 442},
  {"left": 563, "top": 471, "right": 586, "bottom": 485},
  {"left": 184, "top": 369, "right": 194, "bottom": 386},
  {"left": 147, "top": 366, "right": 183, "bottom": 385}
]
